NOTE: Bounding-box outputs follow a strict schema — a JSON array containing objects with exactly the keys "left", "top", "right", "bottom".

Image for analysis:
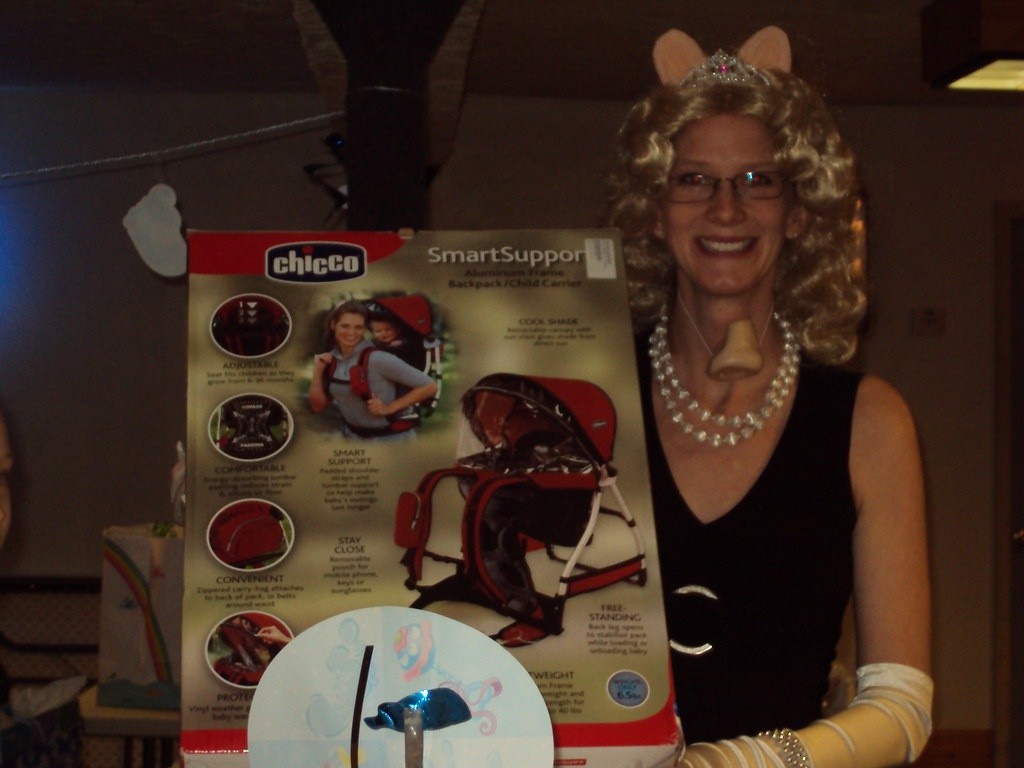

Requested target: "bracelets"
[{"left": 757, "top": 728, "right": 811, "bottom": 768}]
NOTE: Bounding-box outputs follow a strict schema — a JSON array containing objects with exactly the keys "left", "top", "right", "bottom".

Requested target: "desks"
[{"left": 80, "top": 682, "right": 185, "bottom": 768}]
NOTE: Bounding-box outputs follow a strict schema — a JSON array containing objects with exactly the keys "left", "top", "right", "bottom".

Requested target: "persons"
[
  {"left": 308, "top": 301, "right": 437, "bottom": 435},
  {"left": 601, "top": 26, "right": 935, "bottom": 768},
  {"left": 368, "top": 313, "right": 426, "bottom": 420}
]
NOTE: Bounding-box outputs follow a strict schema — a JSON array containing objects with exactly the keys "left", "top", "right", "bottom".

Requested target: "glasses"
[{"left": 665, "top": 172, "right": 791, "bottom": 203}]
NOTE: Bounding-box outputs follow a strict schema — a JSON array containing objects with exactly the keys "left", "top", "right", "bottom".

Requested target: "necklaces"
[
  {"left": 649, "top": 298, "right": 801, "bottom": 446},
  {"left": 681, "top": 302, "right": 776, "bottom": 380}
]
